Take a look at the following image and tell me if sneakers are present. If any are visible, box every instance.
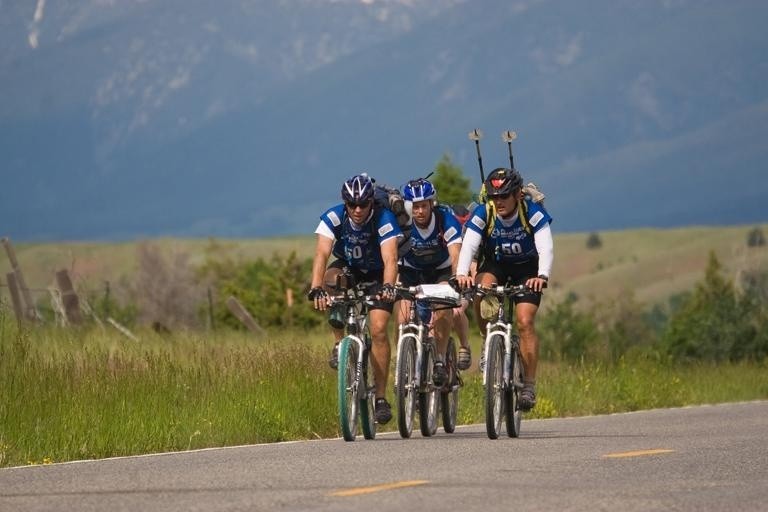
[432,361,446,387]
[521,387,536,404]
[375,398,392,424]
[458,345,472,370]
[330,342,339,368]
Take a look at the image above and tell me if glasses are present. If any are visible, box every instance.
[489,195,509,200]
[348,201,368,209]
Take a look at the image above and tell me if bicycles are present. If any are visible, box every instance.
[382,282,461,439]
[450,275,550,439]
[314,291,393,442]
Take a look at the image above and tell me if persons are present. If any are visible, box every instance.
[390,178,465,395]
[415,267,471,372]
[310,176,403,425]
[454,170,553,407]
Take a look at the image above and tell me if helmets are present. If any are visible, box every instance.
[403,178,436,202]
[342,176,374,205]
[486,168,521,195]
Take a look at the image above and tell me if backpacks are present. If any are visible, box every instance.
[342,185,413,258]
